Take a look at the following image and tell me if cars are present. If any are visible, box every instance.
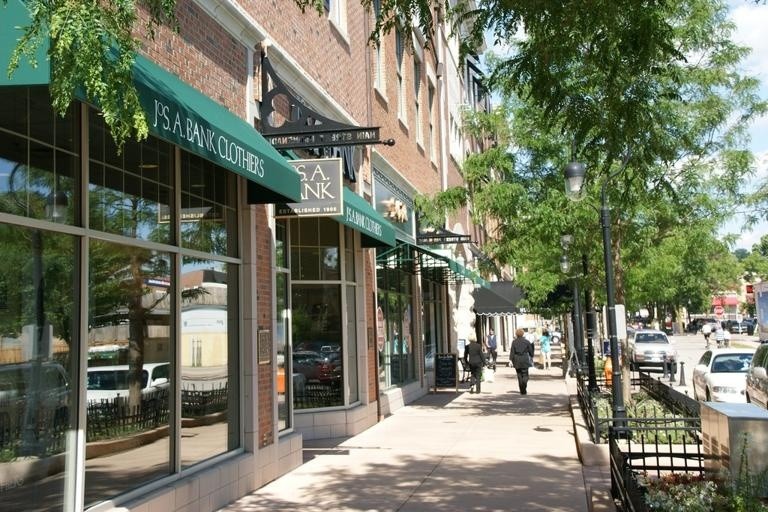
[628,329,677,374]
[692,349,756,404]
[687,319,717,333]
[517,326,562,344]
[293,339,342,387]
[86,358,169,409]
[0,362,70,438]
[746,340,768,411]
[730,323,749,334]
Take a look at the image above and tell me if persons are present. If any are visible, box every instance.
[509,329,533,394]
[464,334,489,393]
[701,322,730,348]
[522,328,534,351]
[539,328,551,369]
[486,328,498,370]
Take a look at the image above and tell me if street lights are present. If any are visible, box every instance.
[562,127,632,439]
[560,229,601,392]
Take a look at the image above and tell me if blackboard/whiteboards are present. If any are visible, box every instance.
[434,353,459,388]
